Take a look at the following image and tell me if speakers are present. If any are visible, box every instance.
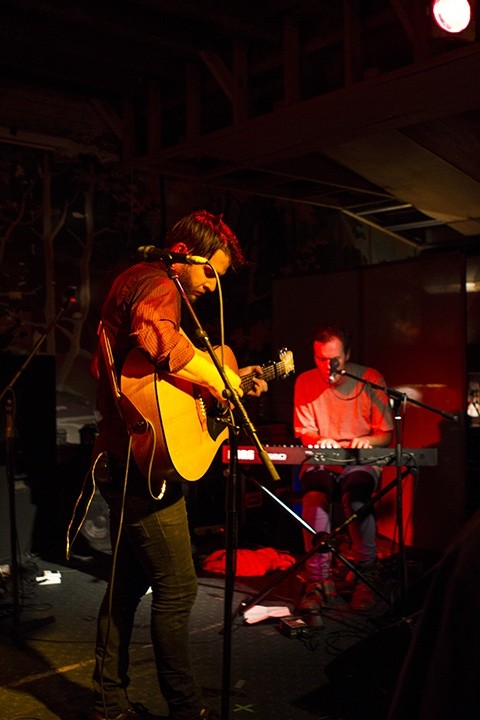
[0,346,114,565]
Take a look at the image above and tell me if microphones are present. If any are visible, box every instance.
[330,358,340,377]
[137,246,208,265]
[63,286,77,303]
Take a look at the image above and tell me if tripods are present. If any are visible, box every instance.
[0,297,70,648]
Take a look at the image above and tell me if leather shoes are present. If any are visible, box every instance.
[300,592,323,610]
[352,572,376,610]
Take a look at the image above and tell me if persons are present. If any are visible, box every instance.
[293,329,394,614]
[91,212,267,720]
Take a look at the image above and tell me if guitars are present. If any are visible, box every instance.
[118,340,297,485]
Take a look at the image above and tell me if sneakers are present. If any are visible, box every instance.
[196,706,224,720]
[94,702,152,720]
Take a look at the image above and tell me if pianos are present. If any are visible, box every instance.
[221,442,433,613]
